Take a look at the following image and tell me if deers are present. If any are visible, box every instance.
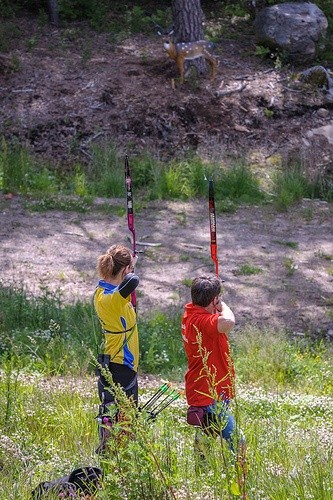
[156,29,217,89]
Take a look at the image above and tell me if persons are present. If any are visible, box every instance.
[180,272,248,478]
[91,242,143,460]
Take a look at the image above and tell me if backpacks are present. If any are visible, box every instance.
[32,462,101,497]
